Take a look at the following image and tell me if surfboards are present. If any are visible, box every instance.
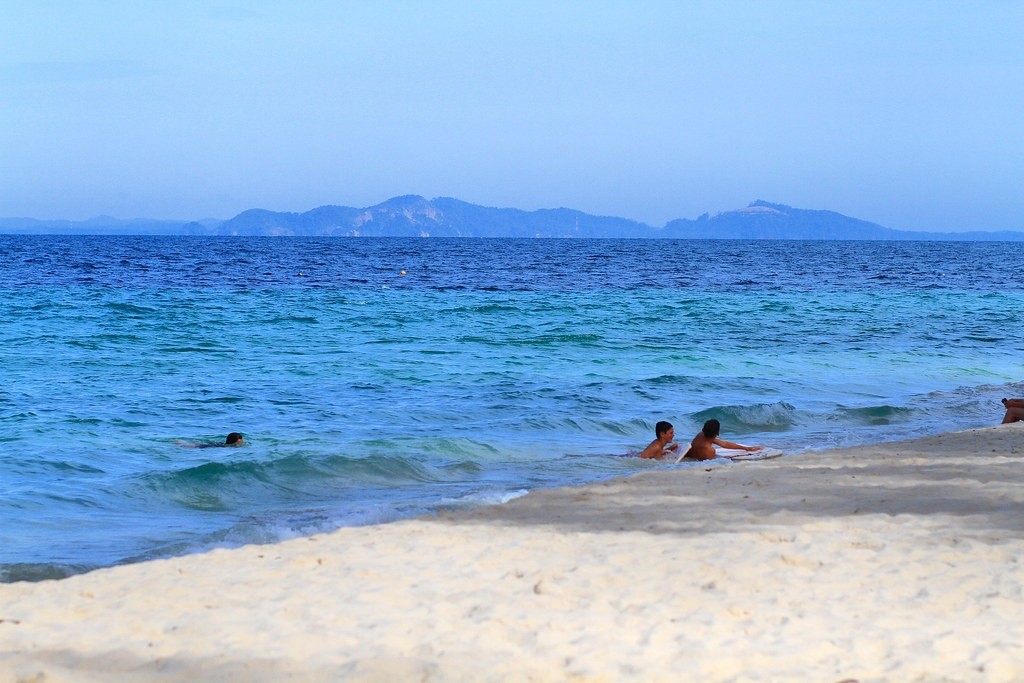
[715,446,783,460]
[666,443,692,466]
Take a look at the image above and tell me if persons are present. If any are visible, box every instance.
[686,419,760,461]
[226,433,242,443]
[641,421,678,459]
[1002,399,1024,423]
